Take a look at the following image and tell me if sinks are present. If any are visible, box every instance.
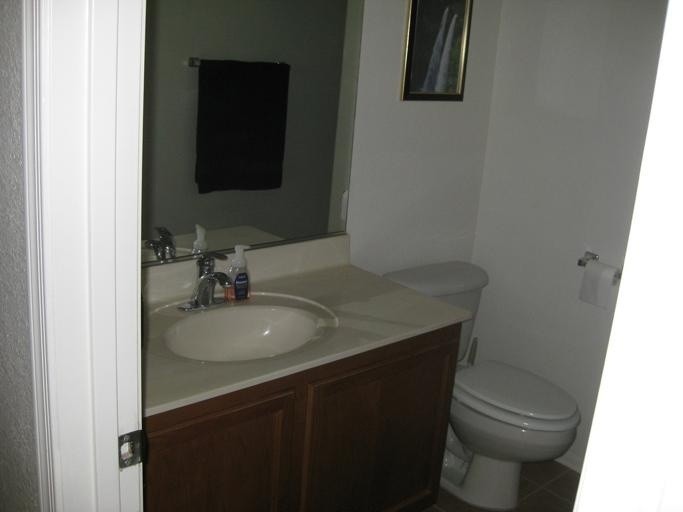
[143,291,340,363]
[140,245,194,265]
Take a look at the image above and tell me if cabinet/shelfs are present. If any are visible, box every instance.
[144,323,460,511]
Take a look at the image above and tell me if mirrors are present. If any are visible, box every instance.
[142,0,366,271]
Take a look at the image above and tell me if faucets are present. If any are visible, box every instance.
[192,250,233,304]
[144,227,177,259]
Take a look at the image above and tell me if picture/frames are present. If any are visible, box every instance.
[400,0,472,101]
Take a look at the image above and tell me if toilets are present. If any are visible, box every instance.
[384,263,581,511]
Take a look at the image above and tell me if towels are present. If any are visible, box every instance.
[196,59,291,195]
